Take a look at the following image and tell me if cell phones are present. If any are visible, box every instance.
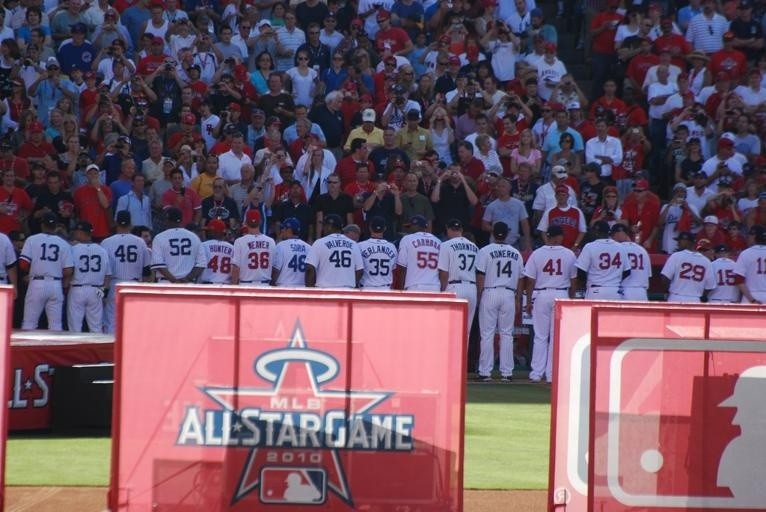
[180,186,185,197]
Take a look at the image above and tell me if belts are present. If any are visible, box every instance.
[447,279,604,294]
[0,274,272,288]
[358,282,394,288]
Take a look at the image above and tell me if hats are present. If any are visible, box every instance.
[0,0,765,252]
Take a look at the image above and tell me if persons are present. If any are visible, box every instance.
[1,1,766,385]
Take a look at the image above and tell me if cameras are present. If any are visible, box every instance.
[114,144,124,148]
[263,178,272,182]
[507,97,513,102]
[386,185,393,189]
[689,109,699,114]
[211,84,220,90]
[396,97,405,106]
[98,88,109,104]
[450,170,460,177]
[23,56,32,66]
[632,128,640,133]
